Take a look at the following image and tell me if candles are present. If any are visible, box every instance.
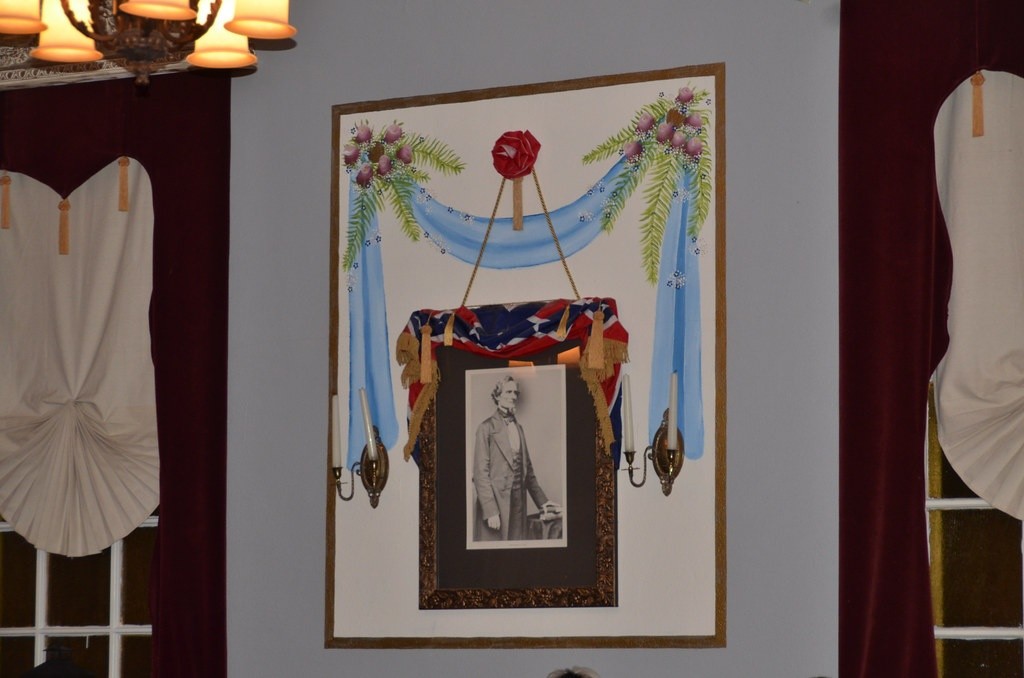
[359,387,377,459]
[667,370,679,449]
[332,394,340,467]
[622,374,635,451]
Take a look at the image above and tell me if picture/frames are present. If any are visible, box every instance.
[400,296,630,609]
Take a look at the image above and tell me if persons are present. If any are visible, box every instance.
[472,376,560,541]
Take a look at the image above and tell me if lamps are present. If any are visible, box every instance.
[0,0,296,85]
